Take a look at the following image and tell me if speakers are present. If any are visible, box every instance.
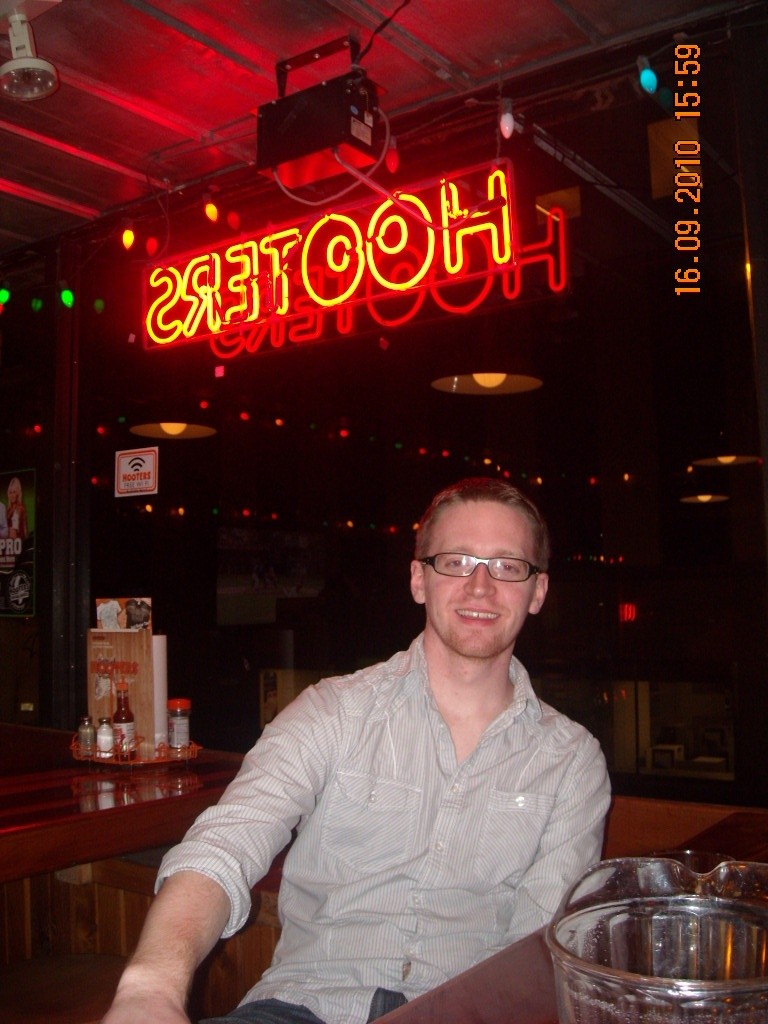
[254,72,378,190]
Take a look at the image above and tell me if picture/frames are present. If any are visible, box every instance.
[0,468,37,619]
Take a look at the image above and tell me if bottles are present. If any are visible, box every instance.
[79,718,95,756]
[113,683,136,760]
[168,699,191,748]
[97,718,114,758]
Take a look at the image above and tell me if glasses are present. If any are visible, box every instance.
[420,551,545,582]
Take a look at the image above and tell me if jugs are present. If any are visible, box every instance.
[544,857,768,1024]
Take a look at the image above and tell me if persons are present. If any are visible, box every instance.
[100,477,612,1024]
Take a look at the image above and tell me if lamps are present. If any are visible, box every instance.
[431,327,543,395]
[674,476,731,505]
[1,8,58,101]
[128,396,218,438]
[687,429,761,468]
